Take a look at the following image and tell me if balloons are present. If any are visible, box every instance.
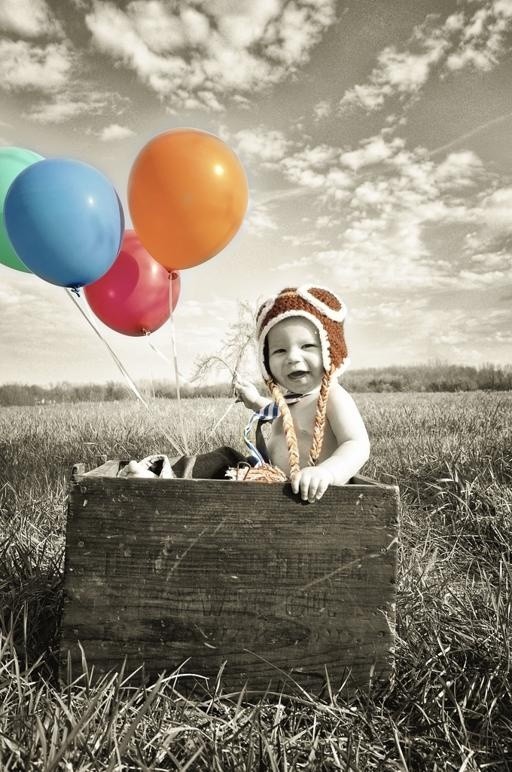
[4,157,125,286]
[84,230,181,336]
[128,130,246,272]
[0,145,46,272]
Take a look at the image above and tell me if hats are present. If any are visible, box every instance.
[256,288,348,383]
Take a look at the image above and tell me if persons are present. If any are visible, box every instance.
[232,285,370,505]
